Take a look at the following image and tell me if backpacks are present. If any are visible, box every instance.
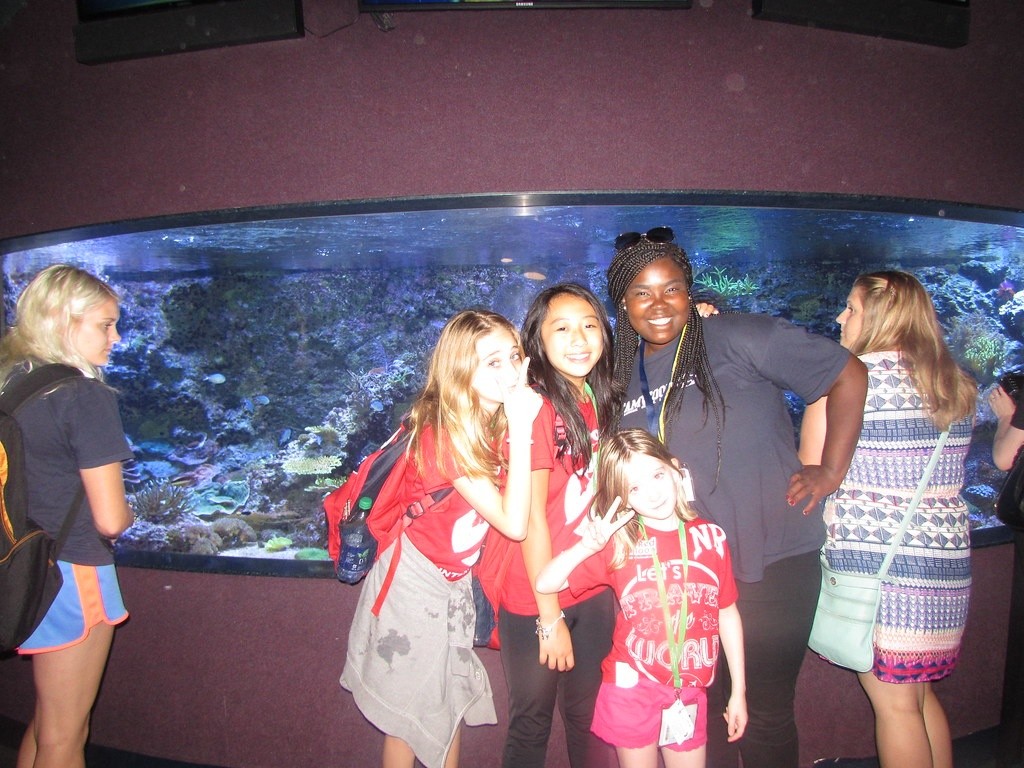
[471,541,502,652]
[323,417,453,584]
[1,363,86,652]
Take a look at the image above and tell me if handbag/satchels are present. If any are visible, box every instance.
[807,555,883,672]
[994,445,1024,534]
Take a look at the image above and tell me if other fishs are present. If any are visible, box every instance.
[279,428,292,445]
[253,395,269,405]
[202,373,226,385]
[370,401,384,412]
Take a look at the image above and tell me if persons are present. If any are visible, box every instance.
[497,283,721,767]
[985,372,1024,767]
[799,270,980,768]
[534,427,749,768]
[339,308,545,767]
[0,261,135,768]
[600,233,870,768]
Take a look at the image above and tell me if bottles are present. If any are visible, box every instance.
[335,496,374,585]
[470,575,491,646]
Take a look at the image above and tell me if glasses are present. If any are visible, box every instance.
[614,226,674,250]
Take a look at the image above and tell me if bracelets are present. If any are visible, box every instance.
[534,608,565,640]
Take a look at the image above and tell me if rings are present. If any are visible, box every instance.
[989,396,995,403]
[994,393,1000,399]
